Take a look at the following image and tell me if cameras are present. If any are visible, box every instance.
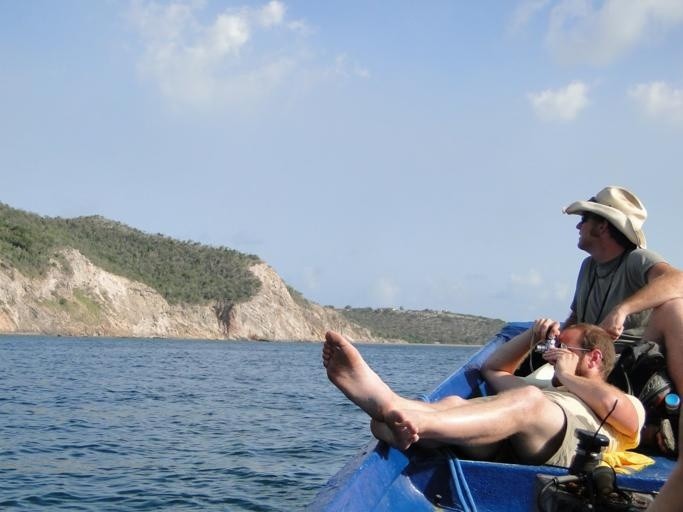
[534,335,557,354]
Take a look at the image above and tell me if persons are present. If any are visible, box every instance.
[563,187,683,453]
[322,318,647,469]
[643,405,683,512]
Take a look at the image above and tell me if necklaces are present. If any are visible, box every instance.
[594,263,617,279]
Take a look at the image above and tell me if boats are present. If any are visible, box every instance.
[298,321,678,512]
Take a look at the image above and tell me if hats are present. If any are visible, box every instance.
[563,186,648,249]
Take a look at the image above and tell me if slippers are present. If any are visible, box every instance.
[646,418,675,457]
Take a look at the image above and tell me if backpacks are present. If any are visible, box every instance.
[607,340,674,417]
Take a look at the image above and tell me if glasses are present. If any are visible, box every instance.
[560,343,592,353]
